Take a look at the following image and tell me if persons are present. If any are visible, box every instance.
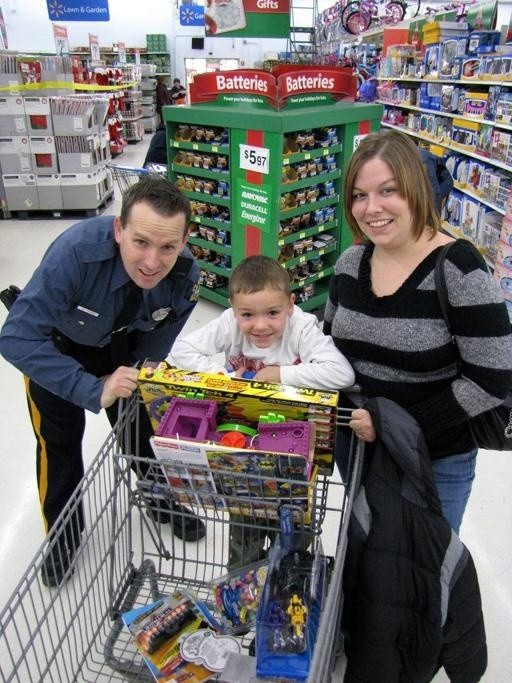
[142,123,167,169]
[0,176,207,588]
[170,78,188,105]
[155,75,172,123]
[169,254,357,658]
[323,127,512,539]
[408,134,454,224]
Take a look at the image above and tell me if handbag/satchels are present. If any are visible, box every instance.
[470,405,512,452]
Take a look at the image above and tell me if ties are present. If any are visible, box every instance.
[111,280,142,342]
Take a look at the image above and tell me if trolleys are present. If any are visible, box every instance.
[2,358,368,681]
[106,160,167,209]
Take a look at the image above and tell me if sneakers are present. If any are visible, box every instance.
[42,534,82,587]
[146,505,206,542]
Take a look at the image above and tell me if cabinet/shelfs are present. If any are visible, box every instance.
[164,76,510,318]
